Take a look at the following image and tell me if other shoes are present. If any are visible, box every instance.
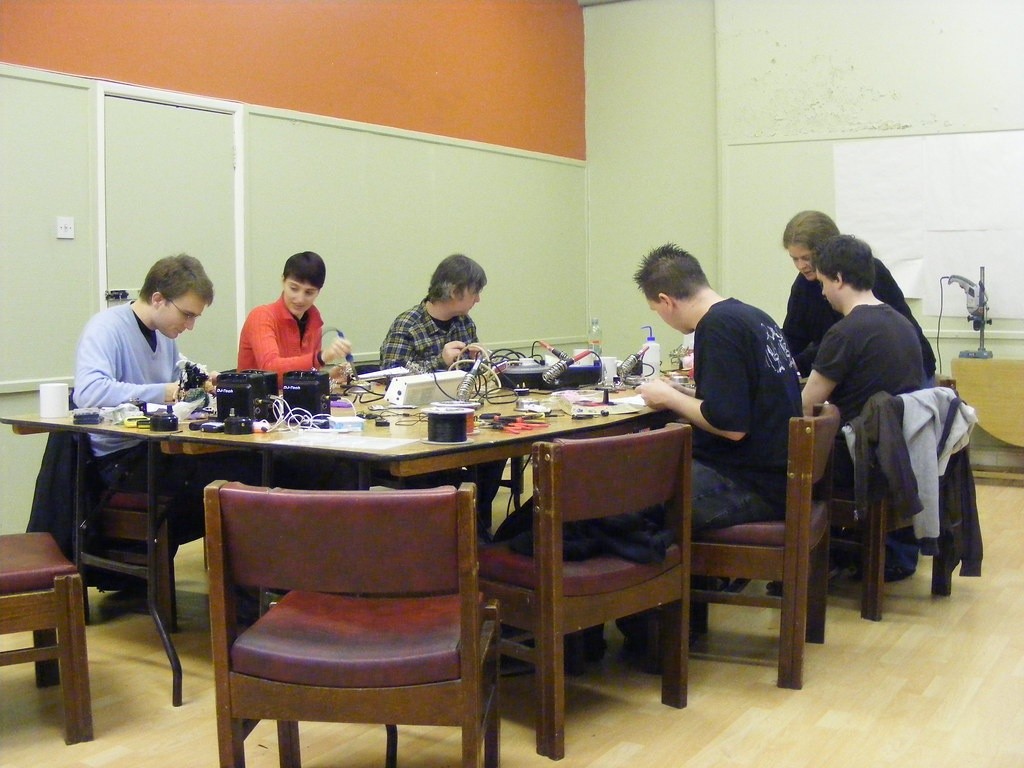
[564,627,609,664]
[618,635,665,674]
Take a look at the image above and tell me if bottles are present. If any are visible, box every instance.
[642,336,661,379]
[587,318,603,356]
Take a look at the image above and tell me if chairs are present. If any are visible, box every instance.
[647,403,841,690]
[23,386,208,632]
[477,422,693,761]
[0,532,94,745]
[204,480,503,768]
[832,376,982,622]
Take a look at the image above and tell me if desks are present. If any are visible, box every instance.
[0,359,812,768]
[950,357,1024,480]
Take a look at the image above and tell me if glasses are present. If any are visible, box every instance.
[169,300,201,320]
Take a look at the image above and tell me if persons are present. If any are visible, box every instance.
[766,234,924,593]
[379,252,508,548]
[73,251,258,624]
[782,211,937,581]
[564,243,802,668]
[236,251,351,389]
